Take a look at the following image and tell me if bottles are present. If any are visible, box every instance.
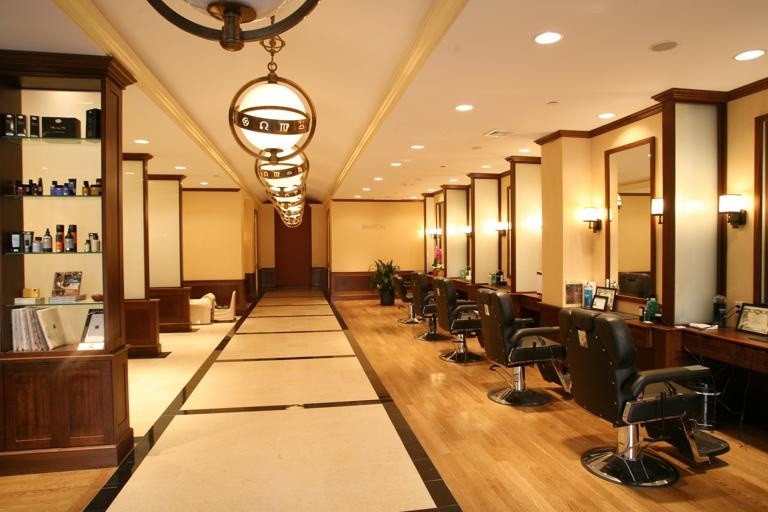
[29,178,101,196]
[25,222,78,253]
[720,307,728,328]
[10,231,21,253]
[654,313,663,322]
[89,232,98,241]
[639,307,644,321]
[84,240,90,252]
[584,282,593,310]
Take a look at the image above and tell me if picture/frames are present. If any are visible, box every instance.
[591,295,609,312]
[596,286,617,312]
[563,280,584,308]
[736,303,768,337]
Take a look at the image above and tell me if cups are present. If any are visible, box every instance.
[91,240,100,253]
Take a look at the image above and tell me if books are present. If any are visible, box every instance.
[11,307,67,351]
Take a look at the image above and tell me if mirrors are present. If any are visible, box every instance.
[435,201,445,272]
[506,185,512,280]
[602,136,657,309]
[751,113,768,309]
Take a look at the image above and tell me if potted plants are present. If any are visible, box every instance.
[367,257,404,307]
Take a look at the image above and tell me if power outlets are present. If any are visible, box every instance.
[733,301,743,312]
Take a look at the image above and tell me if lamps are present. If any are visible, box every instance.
[717,193,748,229]
[228,11,317,166]
[581,207,603,234]
[265,180,306,228]
[255,144,310,197]
[649,197,664,226]
[145,0,321,52]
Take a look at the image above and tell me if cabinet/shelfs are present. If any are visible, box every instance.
[0,48,135,477]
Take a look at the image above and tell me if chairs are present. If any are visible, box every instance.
[556,305,734,491]
[431,275,491,365]
[472,286,577,407]
[408,271,450,343]
[391,274,427,325]
